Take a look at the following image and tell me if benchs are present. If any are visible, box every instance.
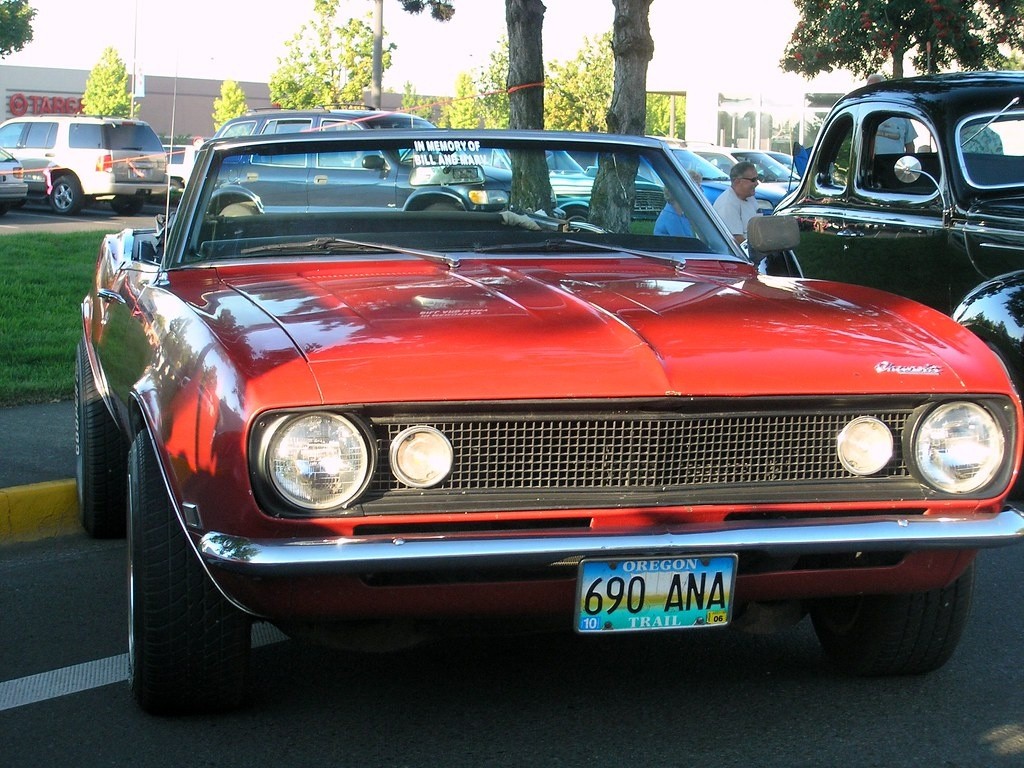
[874,152,1024,190]
[186,205,540,254]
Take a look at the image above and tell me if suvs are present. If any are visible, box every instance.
[0,114,168,215]
[205,104,513,218]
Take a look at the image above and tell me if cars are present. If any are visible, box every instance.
[771,70,1024,407]
[74,128,1024,719]
[0,146,29,215]
[162,145,200,192]
[471,136,801,223]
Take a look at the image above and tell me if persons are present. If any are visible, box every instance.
[961,123,1004,155]
[713,163,791,278]
[653,169,704,239]
[873,117,918,154]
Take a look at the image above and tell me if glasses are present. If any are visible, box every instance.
[740,177,757,182]
